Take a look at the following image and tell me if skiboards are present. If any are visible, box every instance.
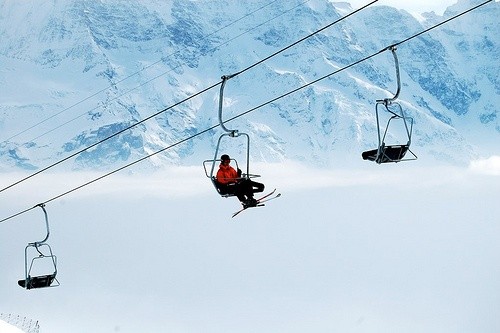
[231,188,282,219]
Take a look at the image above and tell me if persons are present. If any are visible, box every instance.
[215,155,258,208]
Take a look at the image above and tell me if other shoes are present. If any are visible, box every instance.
[242,198,257,209]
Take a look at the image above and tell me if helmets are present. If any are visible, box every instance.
[221,154,230,164]
